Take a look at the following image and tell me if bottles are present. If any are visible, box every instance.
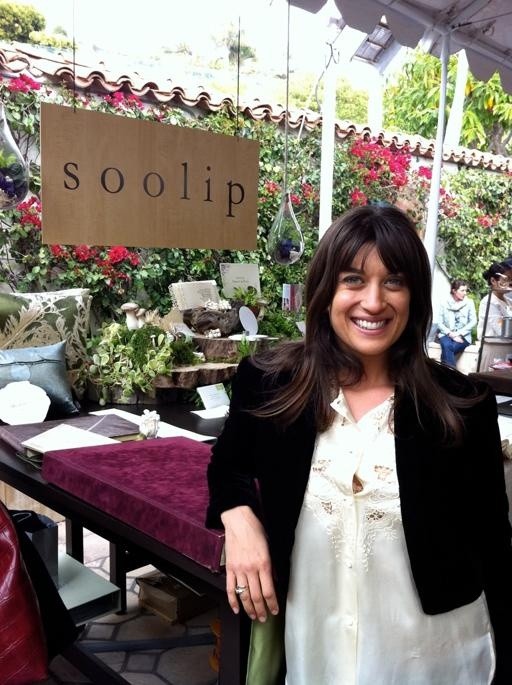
[501,315,512,339]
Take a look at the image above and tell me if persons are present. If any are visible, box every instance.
[201,193,512,684]
[479,262,512,348]
[437,277,477,369]
[500,257,512,303]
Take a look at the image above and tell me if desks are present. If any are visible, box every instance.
[1,399,283,685]
[32,550,129,684]
[470,365,512,425]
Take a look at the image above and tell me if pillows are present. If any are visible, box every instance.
[0,289,95,413]
[0,338,76,419]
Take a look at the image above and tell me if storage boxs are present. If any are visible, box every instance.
[132,569,214,624]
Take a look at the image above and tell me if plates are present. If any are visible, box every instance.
[238,306,259,334]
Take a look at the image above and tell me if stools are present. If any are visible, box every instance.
[428,342,479,373]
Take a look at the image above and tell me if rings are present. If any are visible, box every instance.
[234,585,248,594]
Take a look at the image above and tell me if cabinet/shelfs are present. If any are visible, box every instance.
[474,287,512,374]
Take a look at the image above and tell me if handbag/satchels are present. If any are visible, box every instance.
[9,509,60,591]
